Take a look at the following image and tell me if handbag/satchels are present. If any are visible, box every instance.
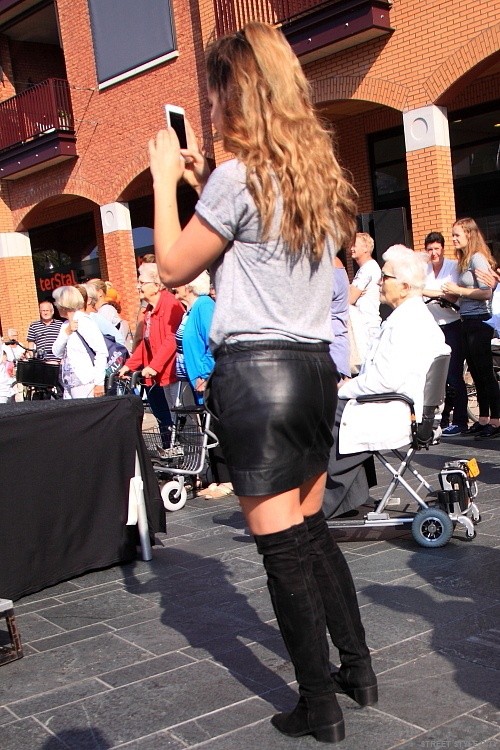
[75,316,130,378]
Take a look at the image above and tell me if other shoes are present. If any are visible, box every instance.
[195,485,216,497]
[204,484,235,500]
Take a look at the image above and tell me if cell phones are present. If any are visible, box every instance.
[165,103,189,153]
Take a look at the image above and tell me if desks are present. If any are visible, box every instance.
[0,395,166,604]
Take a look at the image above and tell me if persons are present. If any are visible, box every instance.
[0,219,500,521]
[148,22,377,743]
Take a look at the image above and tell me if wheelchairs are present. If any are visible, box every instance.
[320,354,481,548]
[103,370,143,396]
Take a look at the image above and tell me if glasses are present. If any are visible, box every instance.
[53,302,59,306]
[136,281,155,285]
[380,272,396,280]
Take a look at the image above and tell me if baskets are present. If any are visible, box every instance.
[140,425,204,471]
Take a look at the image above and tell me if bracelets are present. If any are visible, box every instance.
[460,287,462,297]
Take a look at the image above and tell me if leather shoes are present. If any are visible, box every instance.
[460,421,487,437]
[474,423,500,440]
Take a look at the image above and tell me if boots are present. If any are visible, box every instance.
[252,520,345,743]
[303,507,379,706]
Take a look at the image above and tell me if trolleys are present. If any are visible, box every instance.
[141,380,219,513]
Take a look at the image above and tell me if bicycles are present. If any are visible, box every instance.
[6,339,63,401]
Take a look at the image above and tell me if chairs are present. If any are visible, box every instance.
[36,117,69,134]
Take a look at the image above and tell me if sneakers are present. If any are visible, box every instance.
[441,424,462,436]
